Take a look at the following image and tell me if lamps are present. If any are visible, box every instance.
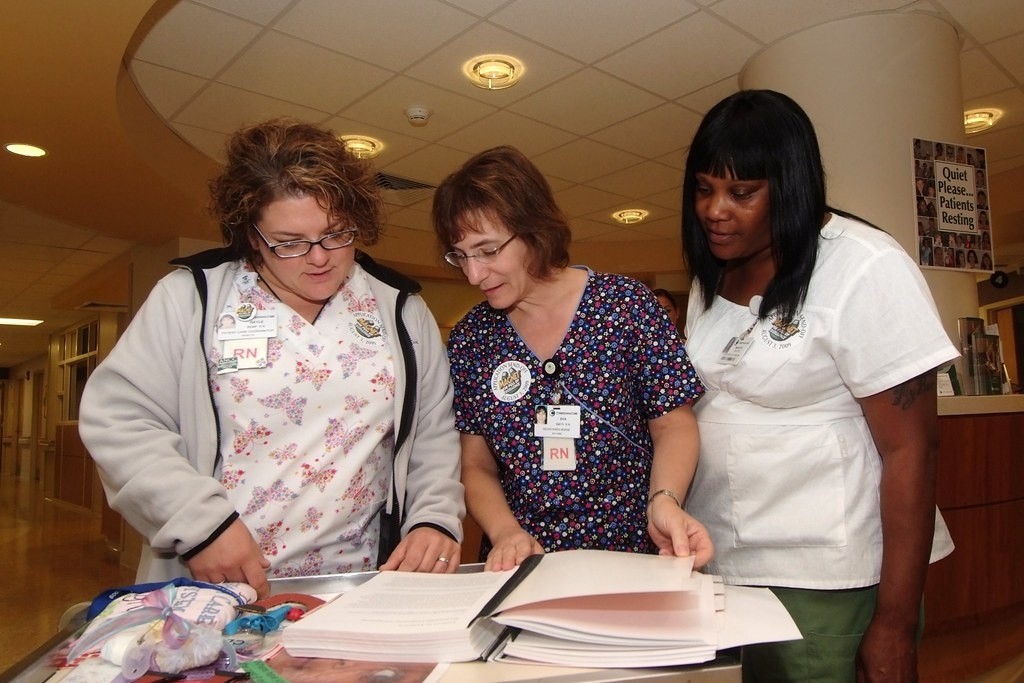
[337,135,385,159]
[964,108,1004,134]
[613,209,648,224]
[462,54,525,90]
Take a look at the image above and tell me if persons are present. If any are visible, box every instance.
[680,89,954,683]
[650,288,680,333]
[912,138,994,271]
[430,146,714,573]
[79,120,467,601]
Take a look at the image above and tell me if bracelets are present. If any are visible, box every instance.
[645,490,681,511]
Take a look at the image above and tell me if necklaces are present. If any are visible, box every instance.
[248,252,341,326]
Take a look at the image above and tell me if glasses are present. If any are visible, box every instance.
[443,233,517,267]
[251,222,359,259]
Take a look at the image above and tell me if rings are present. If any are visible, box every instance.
[437,557,449,566]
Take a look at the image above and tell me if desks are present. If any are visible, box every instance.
[0,563,743,683]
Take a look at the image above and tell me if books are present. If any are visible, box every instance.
[278,551,804,683]
